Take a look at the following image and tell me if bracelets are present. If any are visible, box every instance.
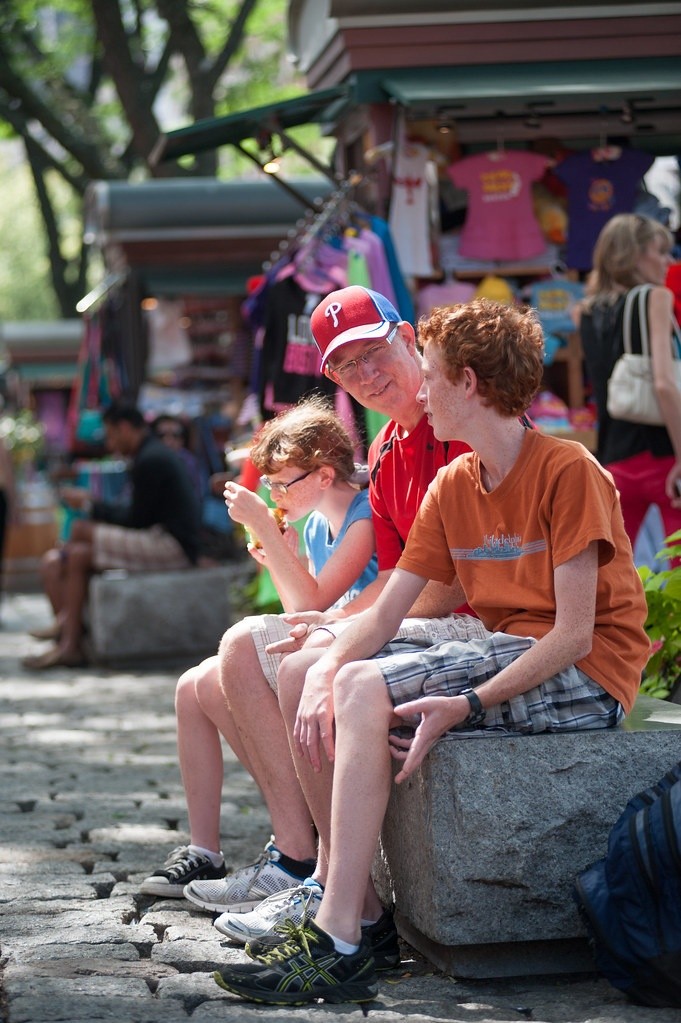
[312,627,336,638]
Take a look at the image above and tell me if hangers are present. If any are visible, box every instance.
[363,135,447,165]
[592,120,621,163]
[489,121,558,167]
[522,258,588,298]
[291,182,369,282]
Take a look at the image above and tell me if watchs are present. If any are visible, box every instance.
[460,688,486,726]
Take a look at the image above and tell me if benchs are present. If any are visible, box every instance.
[366,688,681,978]
[77,568,231,677]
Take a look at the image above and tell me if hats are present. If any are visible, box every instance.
[309,285,402,373]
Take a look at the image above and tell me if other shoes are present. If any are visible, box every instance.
[21,646,82,668]
[28,620,63,645]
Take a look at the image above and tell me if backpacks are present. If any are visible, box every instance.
[570,760,680,1007]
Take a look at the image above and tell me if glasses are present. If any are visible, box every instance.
[157,429,183,438]
[328,325,399,380]
[260,469,315,493]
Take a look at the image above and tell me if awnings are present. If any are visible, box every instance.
[378,57,681,108]
[149,87,349,216]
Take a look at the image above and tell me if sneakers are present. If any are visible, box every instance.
[214,917,378,1005]
[139,846,226,898]
[182,853,306,912]
[214,875,325,942]
[246,904,400,971]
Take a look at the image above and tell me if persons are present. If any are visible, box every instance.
[573,212,681,570]
[22,404,204,671]
[43,415,205,617]
[183,285,535,944]
[139,404,379,897]
[214,298,652,1006]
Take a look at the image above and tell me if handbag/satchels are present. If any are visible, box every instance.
[606,283,681,424]
